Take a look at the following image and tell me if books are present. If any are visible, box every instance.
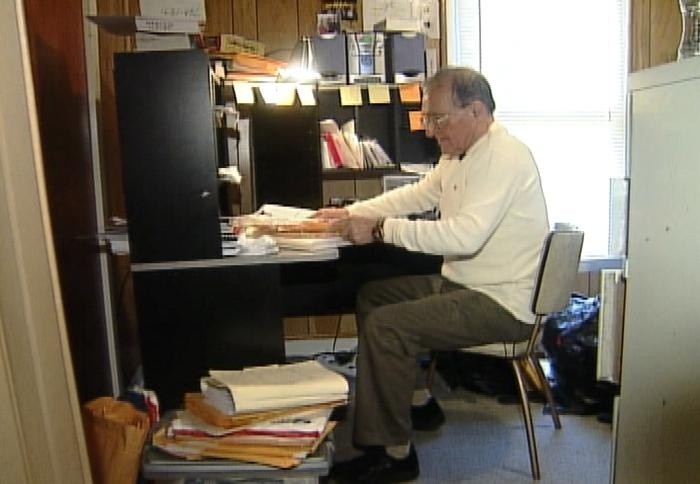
[207,51,280,82]
[95,217,127,241]
[218,203,353,257]
[320,118,392,170]
[151,359,351,471]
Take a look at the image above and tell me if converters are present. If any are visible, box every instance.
[336,352,354,365]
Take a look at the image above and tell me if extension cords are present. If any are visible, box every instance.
[318,354,356,378]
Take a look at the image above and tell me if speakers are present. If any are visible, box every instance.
[308,30,347,83]
[390,30,426,86]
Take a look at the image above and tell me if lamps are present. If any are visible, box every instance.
[263,37,323,87]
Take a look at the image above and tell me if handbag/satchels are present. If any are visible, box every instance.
[81,396,151,484]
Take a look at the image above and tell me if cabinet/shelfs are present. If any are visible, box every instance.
[110,35,451,269]
[589,54,698,481]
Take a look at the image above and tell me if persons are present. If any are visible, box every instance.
[307,67,550,483]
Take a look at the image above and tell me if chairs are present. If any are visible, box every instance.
[454,229,588,480]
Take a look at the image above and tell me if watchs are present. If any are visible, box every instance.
[372,216,384,244]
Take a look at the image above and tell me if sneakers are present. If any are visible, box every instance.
[410,396,445,432]
[319,441,420,484]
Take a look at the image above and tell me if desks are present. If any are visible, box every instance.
[132,218,447,414]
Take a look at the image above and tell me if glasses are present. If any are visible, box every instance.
[419,114,450,129]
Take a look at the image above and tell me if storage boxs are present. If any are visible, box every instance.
[142,442,333,483]
